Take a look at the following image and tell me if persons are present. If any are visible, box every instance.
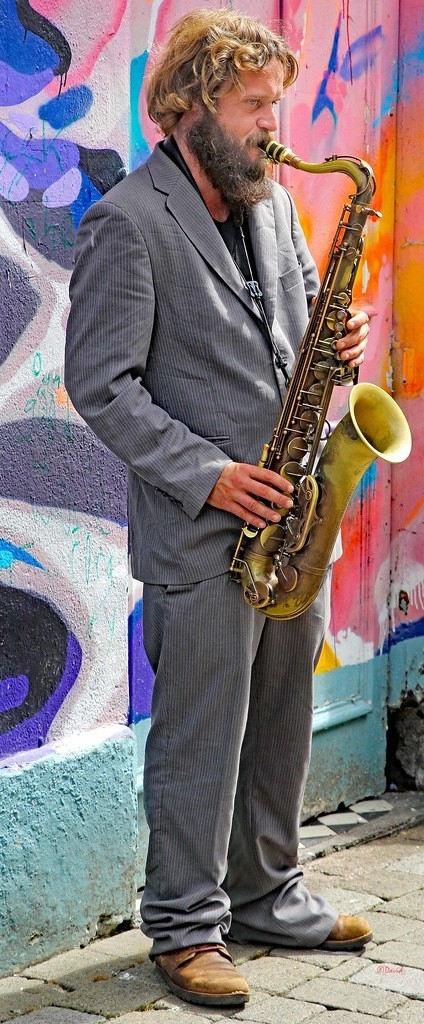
[64,9,374,1005]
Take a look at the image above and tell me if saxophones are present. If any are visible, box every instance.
[222,138,413,619]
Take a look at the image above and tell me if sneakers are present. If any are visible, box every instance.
[152,943,251,1005]
[316,912,374,948]
